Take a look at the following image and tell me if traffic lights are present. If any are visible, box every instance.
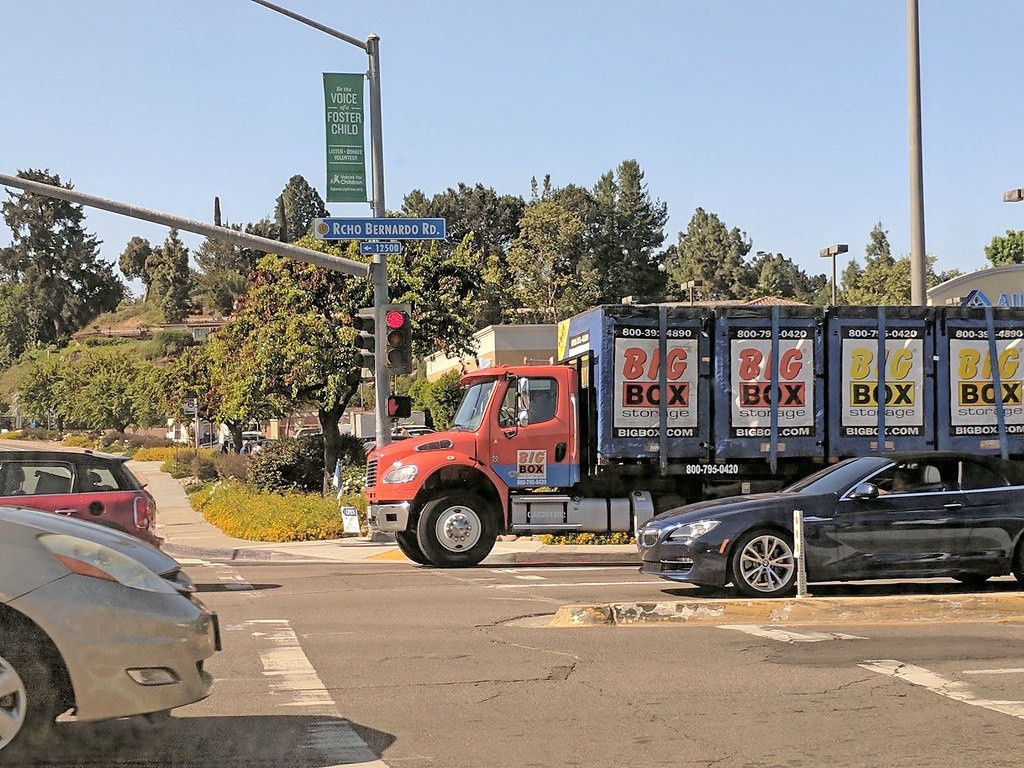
[379,303,412,374]
[352,307,376,379]
[388,395,413,419]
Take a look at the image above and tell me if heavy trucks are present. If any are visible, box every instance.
[365,292,1024,569]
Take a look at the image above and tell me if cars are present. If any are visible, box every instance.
[391,423,436,440]
[240,426,322,457]
[0,442,164,552]
[0,504,223,768]
[634,450,1024,599]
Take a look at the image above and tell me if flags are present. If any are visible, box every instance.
[332,464,345,500]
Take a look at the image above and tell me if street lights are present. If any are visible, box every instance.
[681,280,703,306]
[820,244,849,306]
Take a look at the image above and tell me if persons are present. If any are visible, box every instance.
[874,464,922,495]
[239,440,262,455]
